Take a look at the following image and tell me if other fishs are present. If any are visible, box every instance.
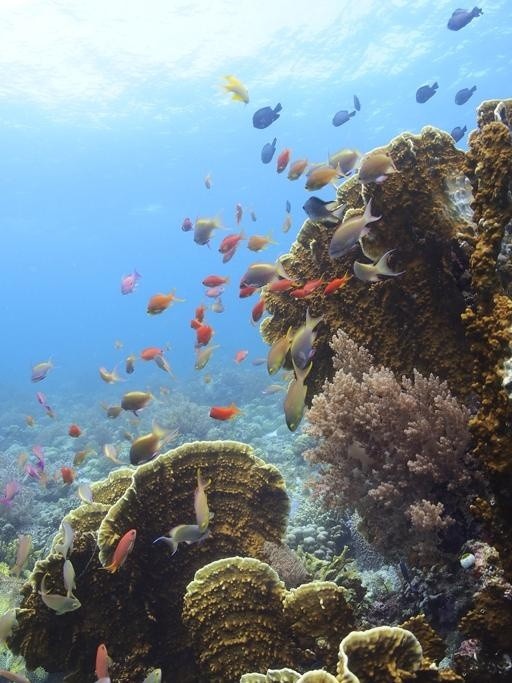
[178,73,406,432]
[0,340,216,639]
